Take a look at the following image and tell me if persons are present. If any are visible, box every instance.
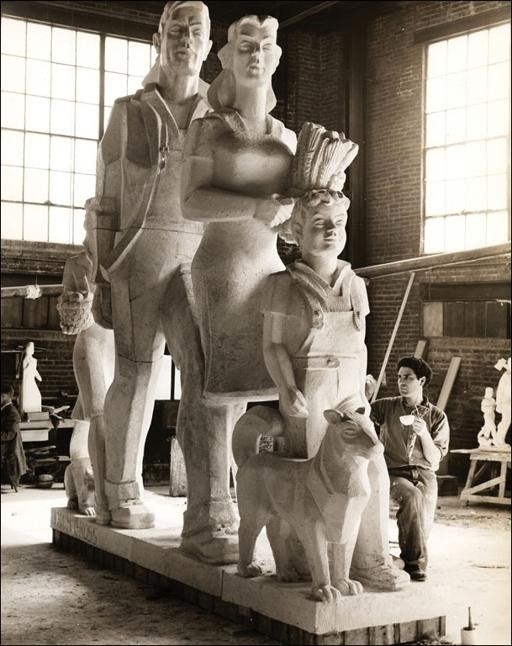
[1,384,30,486]
[366,353,453,582]
[494,357,511,450]
[22,340,44,416]
[479,387,499,439]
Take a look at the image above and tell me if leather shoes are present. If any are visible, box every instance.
[403,565,426,581]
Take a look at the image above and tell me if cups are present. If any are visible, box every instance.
[399,415,415,426]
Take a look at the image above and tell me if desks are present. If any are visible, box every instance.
[461,452,512,508]
